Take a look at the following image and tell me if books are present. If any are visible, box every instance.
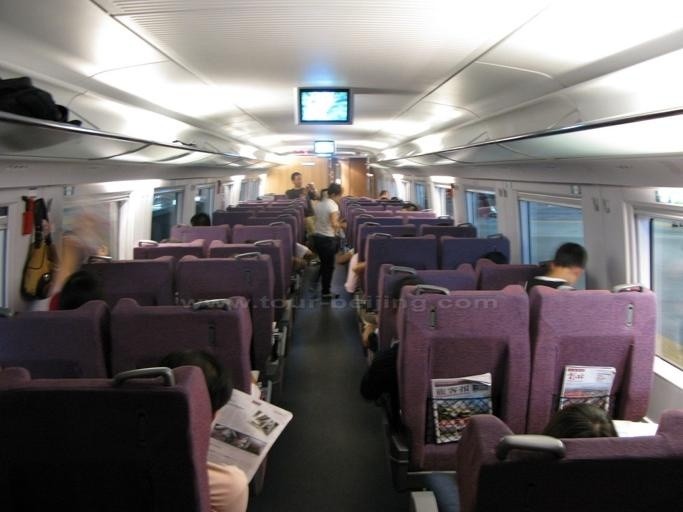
[429,371,494,444]
[560,364,617,414]
[207,387,294,486]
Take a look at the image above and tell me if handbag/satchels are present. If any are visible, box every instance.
[22,200,56,299]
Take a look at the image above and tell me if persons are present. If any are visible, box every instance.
[379,189,389,198]
[403,204,415,211]
[283,172,366,300]
[195,304,262,400]
[422,403,617,511]
[60,271,100,309]
[191,214,212,227]
[170,352,248,512]
[359,344,398,415]
[362,324,377,346]
[525,242,588,290]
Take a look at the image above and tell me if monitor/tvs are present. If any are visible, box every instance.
[314,141,335,154]
[317,154,332,158]
[297,86,353,125]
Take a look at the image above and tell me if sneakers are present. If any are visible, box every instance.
[321,292,340,300]
[309,287,318,299]
